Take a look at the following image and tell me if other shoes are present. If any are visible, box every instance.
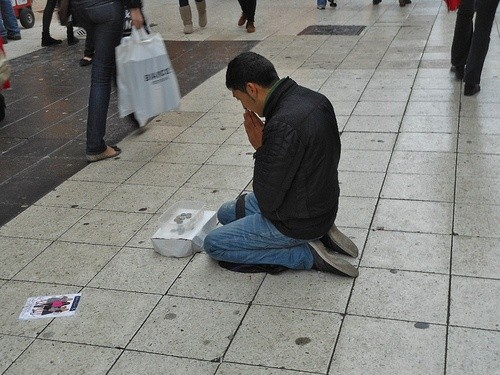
[86,145,122,162]
[317,6,325,10]
[372,0,381,4]
[463,83,480,96]
[307,240,359,278]
[3,33,21,44]
[67,26,79,46]
[79,56,94,67]
[246,21,255,32]
[450,65,464,79]
[320,222,358,258]
[399,0,411,8]
[238,15,246,26]
[42,31,63,46]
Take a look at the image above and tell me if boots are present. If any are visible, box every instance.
[180,5,192,34]
[197,0,207,27]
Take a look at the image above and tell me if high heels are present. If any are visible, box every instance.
[0,93,6,121]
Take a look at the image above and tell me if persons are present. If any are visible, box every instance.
[237,0,258,33]
[203,52,361,279]
[373,0,411,7]
[30,296,74,317]
[450,0,500,96]
[1,0,150,161]
[316,0,337,10]
[178,0,207,34]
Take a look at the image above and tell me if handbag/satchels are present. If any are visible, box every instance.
[115,24,181,128]
[218,193,291,275]
[0,34,12,92]
[445,0,461,12]
